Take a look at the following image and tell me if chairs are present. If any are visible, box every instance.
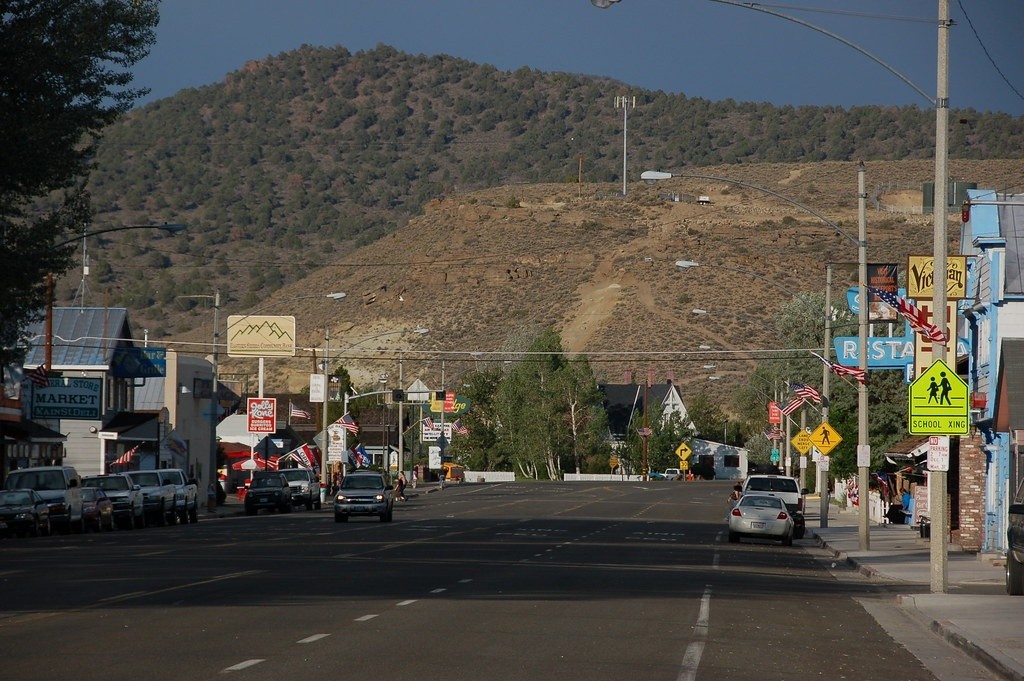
[746,500,754,506]
[771,480,784,490]
[751,479,765,490]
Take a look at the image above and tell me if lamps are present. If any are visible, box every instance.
[178,385,191,395]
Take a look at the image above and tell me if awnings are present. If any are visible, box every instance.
[4,418,67,442]
[98,413,159,440]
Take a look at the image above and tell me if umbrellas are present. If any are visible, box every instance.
[232,458,276,481]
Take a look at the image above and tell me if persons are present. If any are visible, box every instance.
[883,487,911,524]
[412,466,419,488]
[400,471,408,501]
[394,479,405,502]
[438,467,446,489]
[333,471,343,490]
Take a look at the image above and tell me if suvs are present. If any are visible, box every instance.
[333,472,394,523]
[244,470,293,515]
[281,468,322,511]
[741,473,809,539]
[123,470,177,528]
[85,473,146,530]
[157,469,198,524]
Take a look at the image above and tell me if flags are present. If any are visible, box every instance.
[26,366,48,388]
[356,446,371,468]
[866,286,947,342]
[335,413,359,437]
[877,474,889,496]
[790,382,823,404]
[111,445,140,465]
[451,419,468,434]
[348,446,362,469]
[764,426,786,439]
[820,357,865,385]
[290,402,311,420]
[162,431,187,459]
[421,418,433,429]
[290,445,316,476]
[775,398,805,416]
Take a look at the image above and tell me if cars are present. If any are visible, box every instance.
[0,488,51,537]
[728,494,794,546]
[81,486,115,532]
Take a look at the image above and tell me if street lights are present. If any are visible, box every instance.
[640,170,873,550]
[319,328,431,502]
[208,292,347,508]
[675,261,831,527]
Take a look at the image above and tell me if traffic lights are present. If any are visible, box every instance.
[392,388,404,402]
[436,390,446,400]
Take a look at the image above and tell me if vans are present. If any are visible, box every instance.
[7,466,84,534]
[664,468,684,481]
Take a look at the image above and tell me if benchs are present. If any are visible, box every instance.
[900,510,912,516]
